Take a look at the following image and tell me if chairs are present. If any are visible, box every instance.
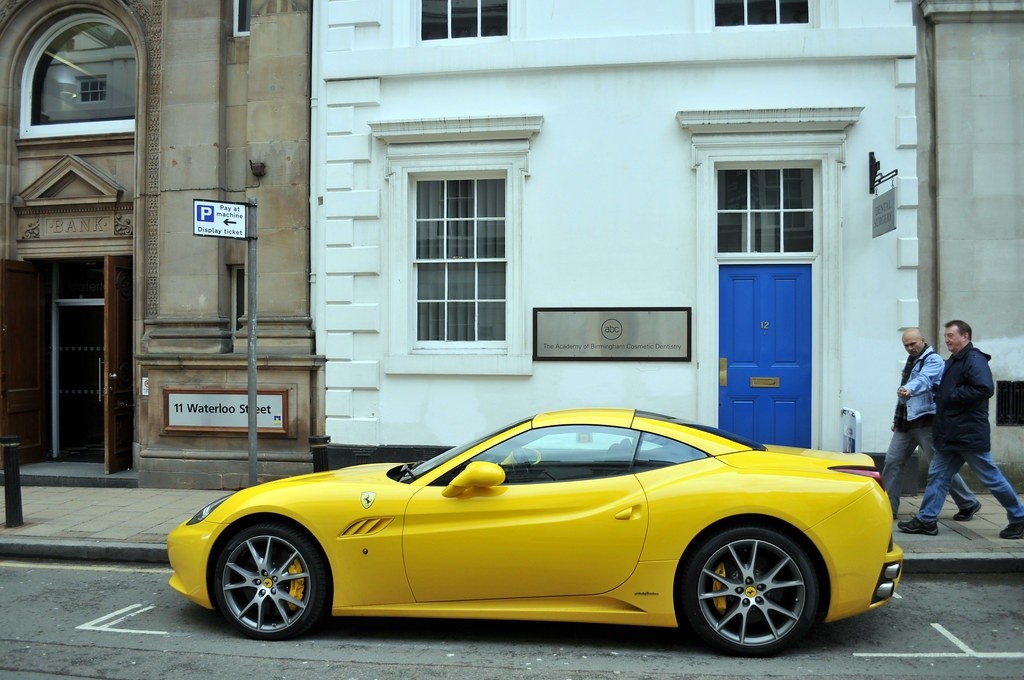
[602,439,634,472]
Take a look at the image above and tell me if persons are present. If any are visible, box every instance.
[881,327,982,521]
[897,320,1024,539]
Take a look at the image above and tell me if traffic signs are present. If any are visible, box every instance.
[192,199,253,243]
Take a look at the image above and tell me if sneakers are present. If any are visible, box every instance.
[953,501,981,521]
[999,520,1024,538]
[898,516,938,535]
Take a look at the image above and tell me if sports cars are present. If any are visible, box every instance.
[166,407,903,661]
